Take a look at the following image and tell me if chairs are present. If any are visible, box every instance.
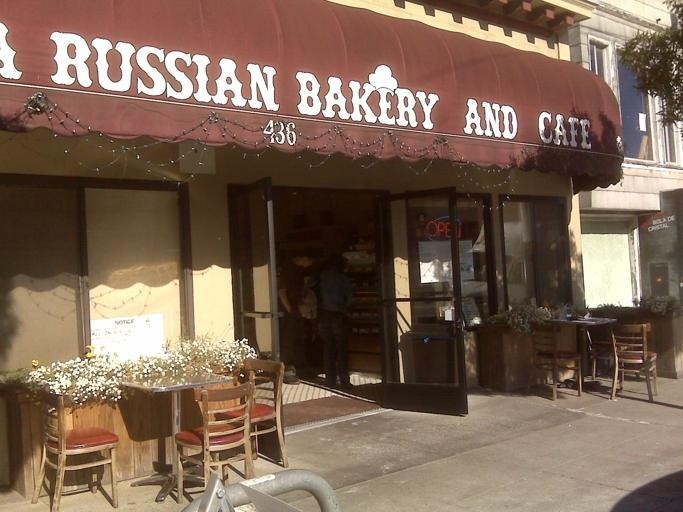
[581,320,630,383]
[215,359,290,473]
[523,323,584,401]
[32,392,118,512]
[608,323,660,403]
[175,381,255,507]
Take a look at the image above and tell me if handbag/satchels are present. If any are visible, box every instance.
[297,288,318,320]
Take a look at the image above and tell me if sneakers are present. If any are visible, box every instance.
[283,367,349,388]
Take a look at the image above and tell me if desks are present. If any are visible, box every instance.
[120,373,238,503]
[560,317,617,386]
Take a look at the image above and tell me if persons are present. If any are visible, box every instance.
[316,252,359,390]
[275,248,316,386]
